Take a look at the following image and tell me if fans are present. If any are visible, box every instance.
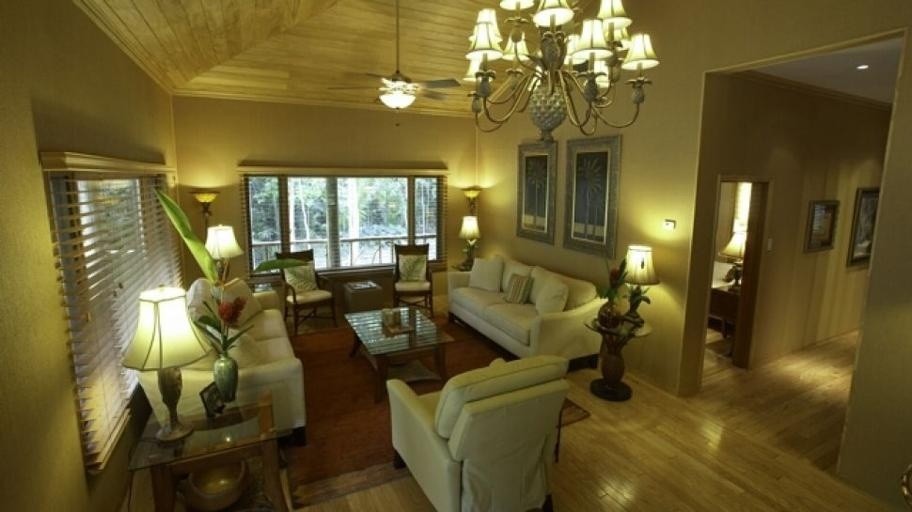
[325,1,460,99]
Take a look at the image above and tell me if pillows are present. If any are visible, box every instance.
[283,260,318,294]
[467,253,503,294]
[503,272,535,305]
[534,276,568,314]
[221,277,263,328]
[204,323,267,367]
[398,254,427,281]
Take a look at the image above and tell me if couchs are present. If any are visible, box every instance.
[139,278,307,448]
[446,253,609,370]
[386,357,566,510]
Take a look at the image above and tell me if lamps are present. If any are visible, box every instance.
[463,0,661,141]
[462,186,482,213]
[375,77,426,129]
[118,289,214,441]
[457,215,480,271]
[722,230,748,290]
[623,247,660,327]
[188,190,221,220]
[205,226,246,282]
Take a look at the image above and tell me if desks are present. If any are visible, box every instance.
[581,323,653,402]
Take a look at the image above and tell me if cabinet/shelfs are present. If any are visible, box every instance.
[342,281,383,311]
[126,390,288,512]
[712,285,742,339]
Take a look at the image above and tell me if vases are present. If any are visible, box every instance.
[598,301,622,328]
[212,350,239,402]
[199,382,225,417]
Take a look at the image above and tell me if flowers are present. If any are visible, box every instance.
[155,186,309,355]
[597,254,629,301]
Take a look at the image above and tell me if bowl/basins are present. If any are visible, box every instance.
[188,453,251,512]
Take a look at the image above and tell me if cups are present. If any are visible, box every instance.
[408,305,417,322]
[382,308,401,326]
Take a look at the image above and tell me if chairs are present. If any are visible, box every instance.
[391,242,434,319]
[274,248,338,338]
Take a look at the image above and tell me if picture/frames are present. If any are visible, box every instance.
[846,185,880,269]
[515,141,557,246]
[562,136,623,262]
[803,199,839,254]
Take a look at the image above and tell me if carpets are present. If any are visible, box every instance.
[283,312,592,510]
